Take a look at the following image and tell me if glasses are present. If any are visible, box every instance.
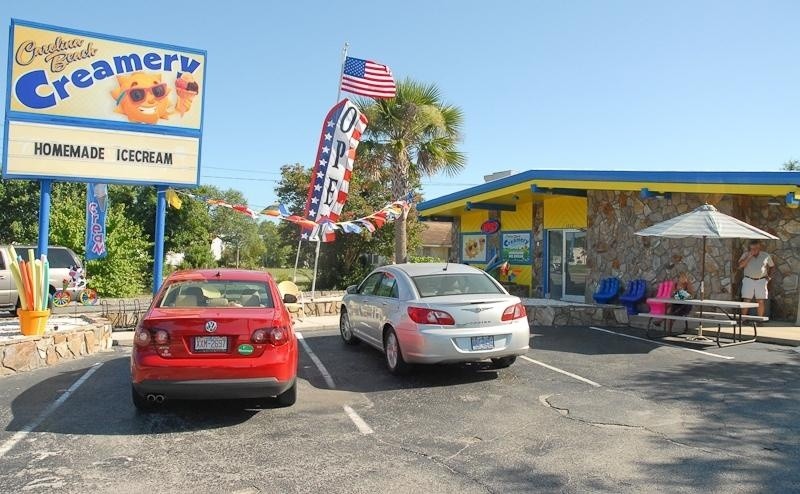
[125,83,167,102]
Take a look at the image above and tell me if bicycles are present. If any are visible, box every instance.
[52,279,97,307]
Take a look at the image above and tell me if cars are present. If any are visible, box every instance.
[131,270,298,409]
[339,262,530,377]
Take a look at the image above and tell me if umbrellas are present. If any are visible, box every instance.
[629,201,780,325]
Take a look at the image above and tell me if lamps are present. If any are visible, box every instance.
[768,198,781,205]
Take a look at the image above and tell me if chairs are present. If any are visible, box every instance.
[279,281,304,322]
[618,279,647,315]
[593,277,619,304]
[646,280,674,313]
[174,291,265,307]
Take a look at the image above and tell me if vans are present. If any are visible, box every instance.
[0,246,86,316]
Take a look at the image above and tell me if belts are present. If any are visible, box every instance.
[745,275,767,280]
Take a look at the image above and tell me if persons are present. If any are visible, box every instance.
[652,270,697,337]
[735,237,776,326]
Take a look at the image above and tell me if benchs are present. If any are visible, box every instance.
[640,313,736,347]
[696,312,769,340]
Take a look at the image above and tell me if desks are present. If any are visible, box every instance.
[651,298,759,343]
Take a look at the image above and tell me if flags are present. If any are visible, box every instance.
[299,98,370,242]
[166,186,414,235]
[340,57,397,100]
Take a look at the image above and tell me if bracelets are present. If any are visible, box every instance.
[768,276,772,280]
